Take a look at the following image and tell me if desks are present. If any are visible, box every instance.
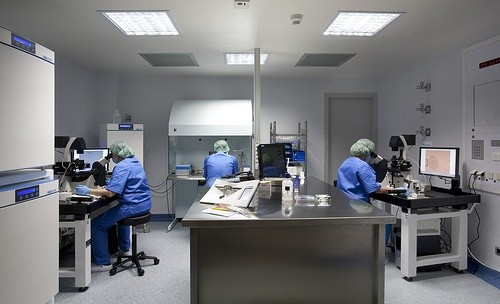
[168,174,246,232]
[59,194,119,292]
[182,176,396,304]
[333,179,480,282]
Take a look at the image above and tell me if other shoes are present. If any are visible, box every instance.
[91,262,113,273]
[117,251,132,257]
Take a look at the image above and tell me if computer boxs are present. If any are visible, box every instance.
[394,234,442,272]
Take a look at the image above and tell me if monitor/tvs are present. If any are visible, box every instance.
[418,147,459,180]
[73,148,110,175]
[257,143,287,179]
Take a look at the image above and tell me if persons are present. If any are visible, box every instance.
[76,139,151,271]
[337,138,392,247]
[203,139,240,182]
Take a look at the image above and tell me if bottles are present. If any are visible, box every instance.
[282,202,292,217]
[113,110,121,123]
[282,173,294,201]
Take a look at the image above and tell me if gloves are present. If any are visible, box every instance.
[76,185,90,196]
[104,178,112,187]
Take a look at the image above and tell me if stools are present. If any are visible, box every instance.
[108,213,159,276]
